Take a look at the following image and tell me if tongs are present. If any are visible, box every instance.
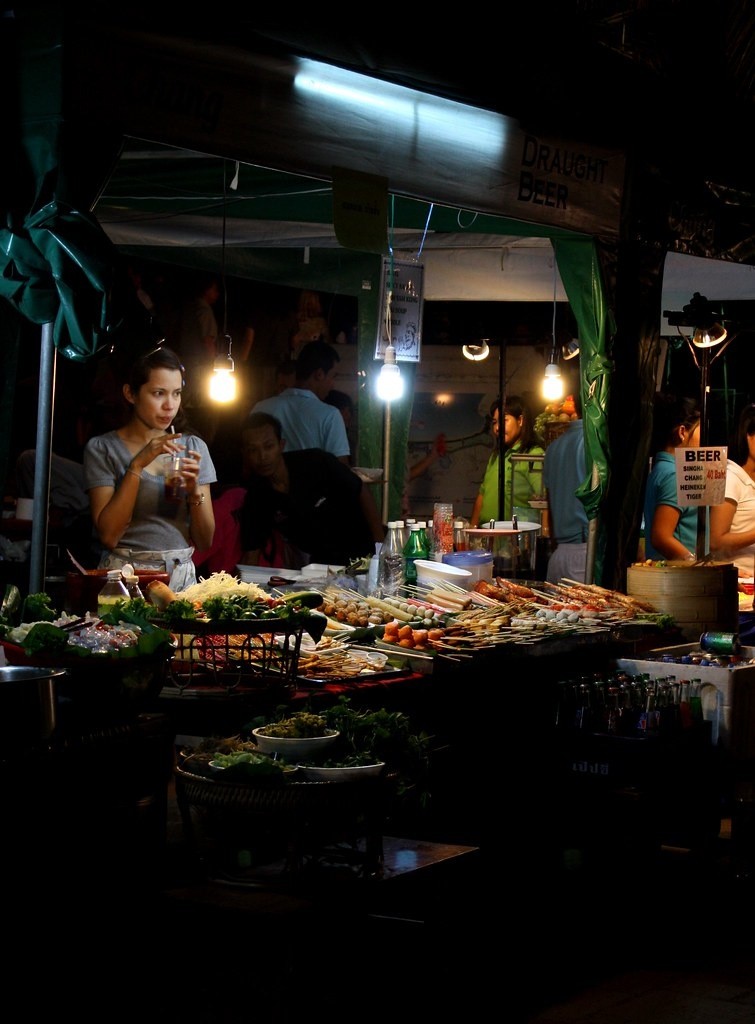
[345,561,369,575]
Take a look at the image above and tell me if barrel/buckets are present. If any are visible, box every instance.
[416,578,468,600]
[465,521,542,579]
[443,552,494,592]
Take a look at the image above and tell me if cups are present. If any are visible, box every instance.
[162,455,190,504]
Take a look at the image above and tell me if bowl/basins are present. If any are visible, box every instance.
[413,559,473,580]
[352,467,383,483]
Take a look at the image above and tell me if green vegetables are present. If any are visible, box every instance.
[211,696,440,813]
[0,581,310,661]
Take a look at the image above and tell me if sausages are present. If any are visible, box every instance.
[427,589,475,610]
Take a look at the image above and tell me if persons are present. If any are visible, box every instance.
[84,347,217,592]
[180,276,219,358]
[191,422,289,573]
[402,445,440,520]
[240,412,382,568]
[544,382,589,582]
[471,395,550,581]
[644,395,710,560]
[291,290,331,343]
[709,403,755,647]
[251,340,353,465]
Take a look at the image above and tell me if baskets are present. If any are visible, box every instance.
[139,610,309,698]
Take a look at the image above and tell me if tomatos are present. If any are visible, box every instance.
[544,395,580,423]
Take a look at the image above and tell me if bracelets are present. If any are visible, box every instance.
[191,493,205,506]
[127,469,142,479]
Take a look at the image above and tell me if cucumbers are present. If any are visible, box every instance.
[285,593,324,610]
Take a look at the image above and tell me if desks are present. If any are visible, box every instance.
[146,834,482,917]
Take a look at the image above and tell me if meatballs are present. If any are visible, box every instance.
[315,597,446,651]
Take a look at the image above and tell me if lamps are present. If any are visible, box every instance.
[457,318,491,362]
[557,327,580,361]
[682,292,727,348]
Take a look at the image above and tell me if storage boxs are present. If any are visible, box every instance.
[609,640,755,756]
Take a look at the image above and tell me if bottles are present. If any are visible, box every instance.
[126,576,145,603]
[428,521,434,544]
[405,519,415,542]
[97,570,131,621]
[377,521,405,600]
[416,521,430,548]
[403,524,429,599]
[549,668,704,741]
[453,521,466,552]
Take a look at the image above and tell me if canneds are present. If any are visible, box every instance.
[699,631,741,654]
